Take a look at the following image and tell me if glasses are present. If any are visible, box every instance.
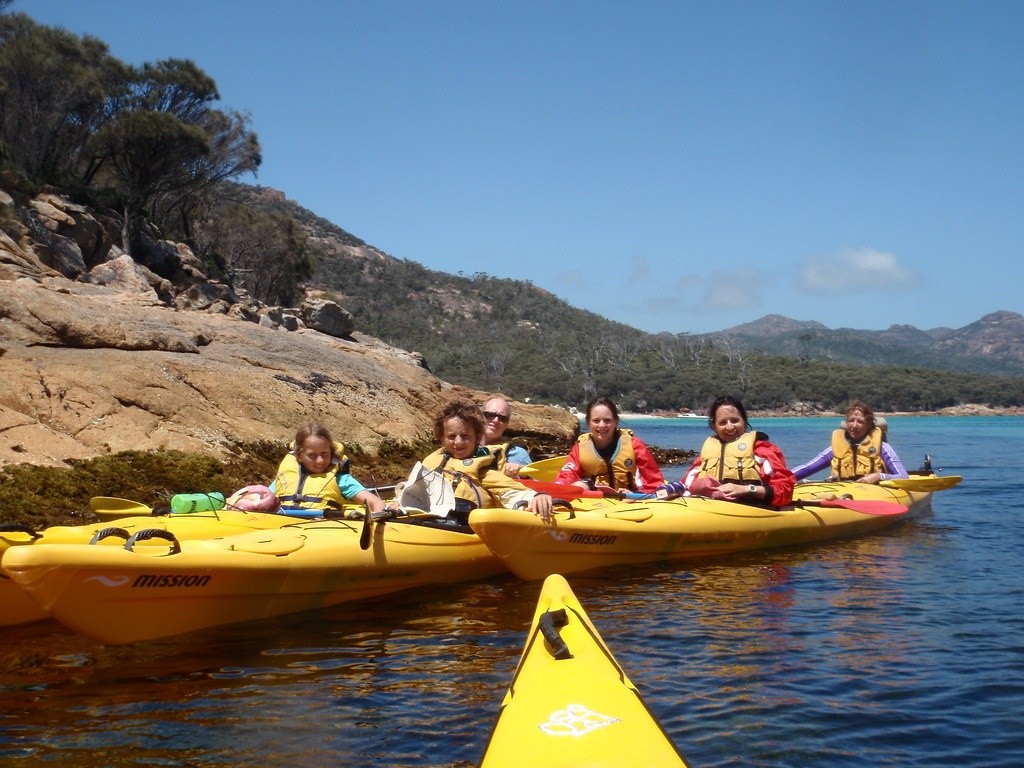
[482,410,510,424]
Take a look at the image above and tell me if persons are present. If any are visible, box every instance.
[552,397,665,493]
[680,395,797,508]
[387,397,554,521]
[268,423,386,514]
[790,399,909,485]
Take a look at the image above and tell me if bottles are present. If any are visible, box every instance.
[655,481,687,501]
[170,490,226,513]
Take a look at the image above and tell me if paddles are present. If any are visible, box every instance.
[784,499,909,515]
[365,455,569,493]
[512,477,656,503]
[90,497,358,523]
[359,499,424,551]
[877,476,961,492]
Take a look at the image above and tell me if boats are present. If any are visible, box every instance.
[482,572,689,768]
[466,456,936,580]
[0,508,515,648]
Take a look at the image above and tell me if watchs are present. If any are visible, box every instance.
[748,484,756,497]
